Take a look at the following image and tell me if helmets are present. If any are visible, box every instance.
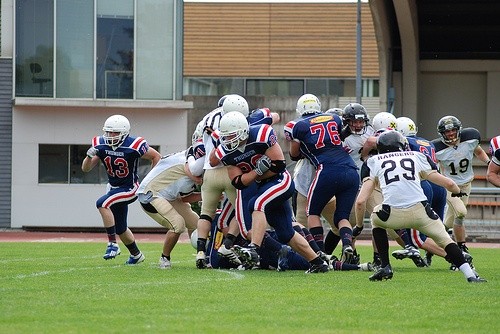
[296,93,321,117]
[102,114,130,142]
[222,94,249,117]
[342,103,370,126]
[191,228,210,250]
[375,129,411,155]
[372,111,397,132]
[218,111,249,140]
[327,107,343,116]
[436,115,463,143]
[397,117,417,137]
[218,94,228,107]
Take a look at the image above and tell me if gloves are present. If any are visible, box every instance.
[451,190,468,198]
[352,225,363,240]
[256,155,271,176]
[86,147,99,159]
[342,145,354,154]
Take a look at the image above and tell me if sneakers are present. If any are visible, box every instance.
[215,245,487,282]
[103,242,121,260]
[124,251,145,265]
[159,256,171,268]
[195,251,206,269]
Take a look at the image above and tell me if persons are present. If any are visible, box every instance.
[135,96,500,284]
[82,114,163,264]
